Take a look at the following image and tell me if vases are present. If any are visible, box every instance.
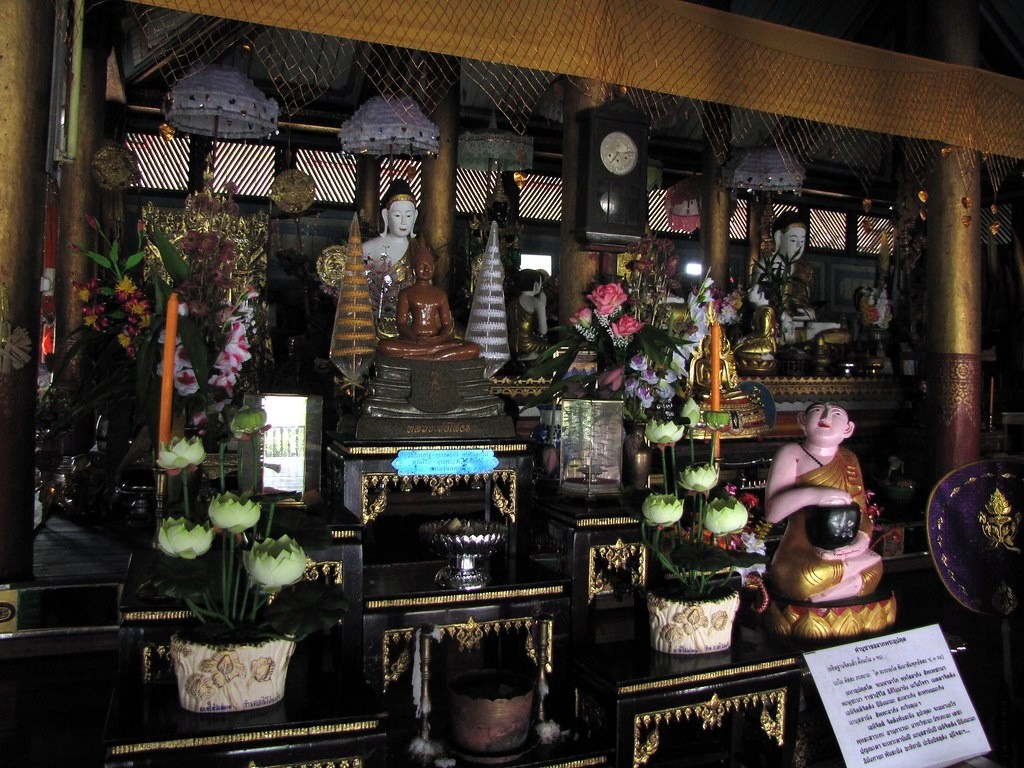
[171,627,296,715]
[143,396,174,455]
[621,421,654,493]
[645,590,742,656]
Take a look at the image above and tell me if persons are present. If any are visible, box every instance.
[765,400,883,600]
[746,210,893,344]
[362,179,564,361]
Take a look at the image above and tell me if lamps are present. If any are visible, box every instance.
[161,62,282,143]
[716,142,806,201]
[336,94,441,164]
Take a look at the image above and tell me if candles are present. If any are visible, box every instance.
[711,318,722,412]
[989,376,995,415]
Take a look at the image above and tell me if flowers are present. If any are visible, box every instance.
[857,188,930,331]
[634,398,772,604]
[134,403,352,643]
[508,231,750,432]
[62,139,272,454]
[745,235,815,344]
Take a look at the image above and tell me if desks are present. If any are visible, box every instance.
[563,598,967,768]
